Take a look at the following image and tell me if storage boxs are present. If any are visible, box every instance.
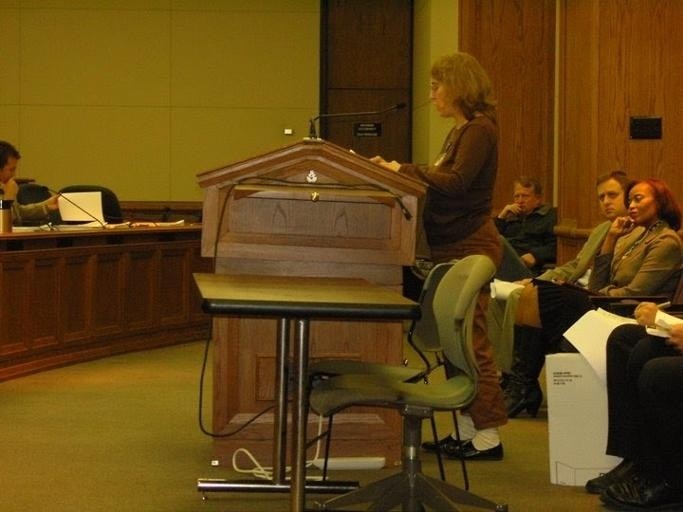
[546,353,623,489]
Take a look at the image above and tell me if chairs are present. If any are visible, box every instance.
[544,243,682,323]
[16,184,49,206]
[56,185,123,224]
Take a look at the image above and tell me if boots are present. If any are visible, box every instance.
[501,323,545,418]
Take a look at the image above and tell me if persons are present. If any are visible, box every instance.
[495,172,560,278]
[498,176,681,418]
[371,50,510,463]
[0,140,60,226]
[487,172,631,377]
[589,301,682,510]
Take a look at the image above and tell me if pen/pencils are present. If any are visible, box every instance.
[631,300,671,317]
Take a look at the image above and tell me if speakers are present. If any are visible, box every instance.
[630,117,661,139]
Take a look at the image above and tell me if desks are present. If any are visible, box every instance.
[192,273,422,511]
[0,221,212,383]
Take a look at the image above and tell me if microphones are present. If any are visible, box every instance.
[42,186,106,229]
[303,102,406,143]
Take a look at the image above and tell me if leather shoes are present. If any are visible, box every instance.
[422,433,468,453]
[448,439,504,461]
[602,470,682,511]
[585,463,633,493]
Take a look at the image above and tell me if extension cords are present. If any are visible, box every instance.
[314,457,385,471]
